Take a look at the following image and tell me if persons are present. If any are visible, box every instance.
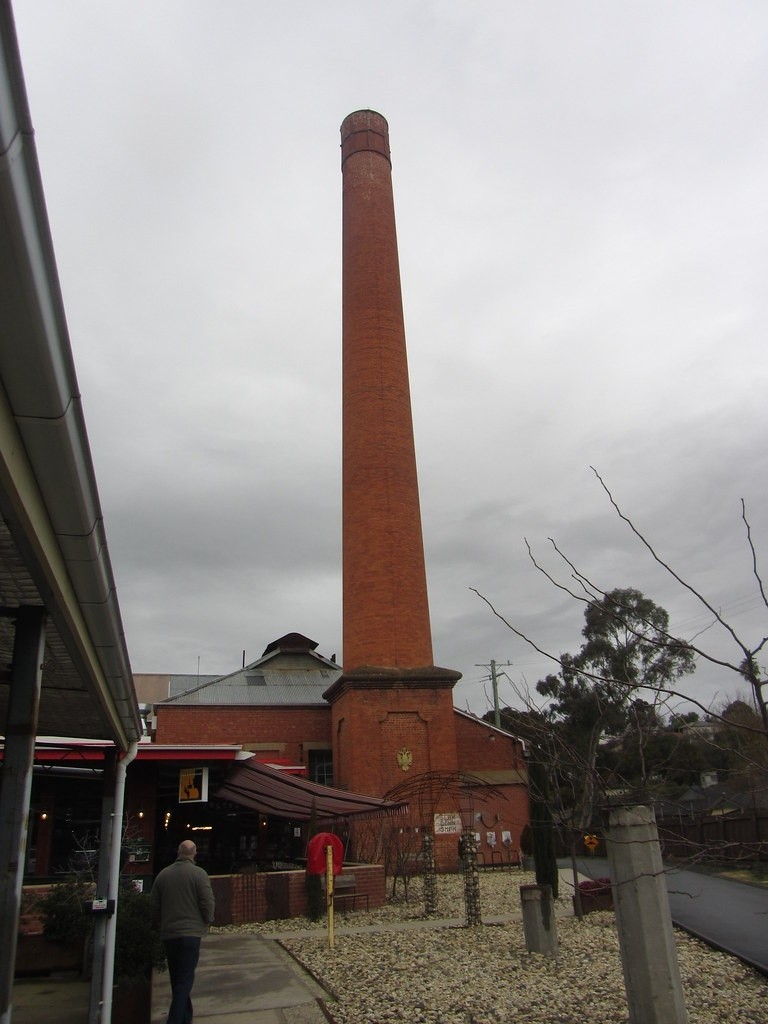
[149,838,215,1024]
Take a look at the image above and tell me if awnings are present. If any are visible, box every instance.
[212,757,409,830]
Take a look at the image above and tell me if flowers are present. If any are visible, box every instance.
[579,877,611,895]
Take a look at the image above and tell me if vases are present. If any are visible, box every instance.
[570,893,613,915]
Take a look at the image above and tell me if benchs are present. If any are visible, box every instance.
[320,874,369,912]
